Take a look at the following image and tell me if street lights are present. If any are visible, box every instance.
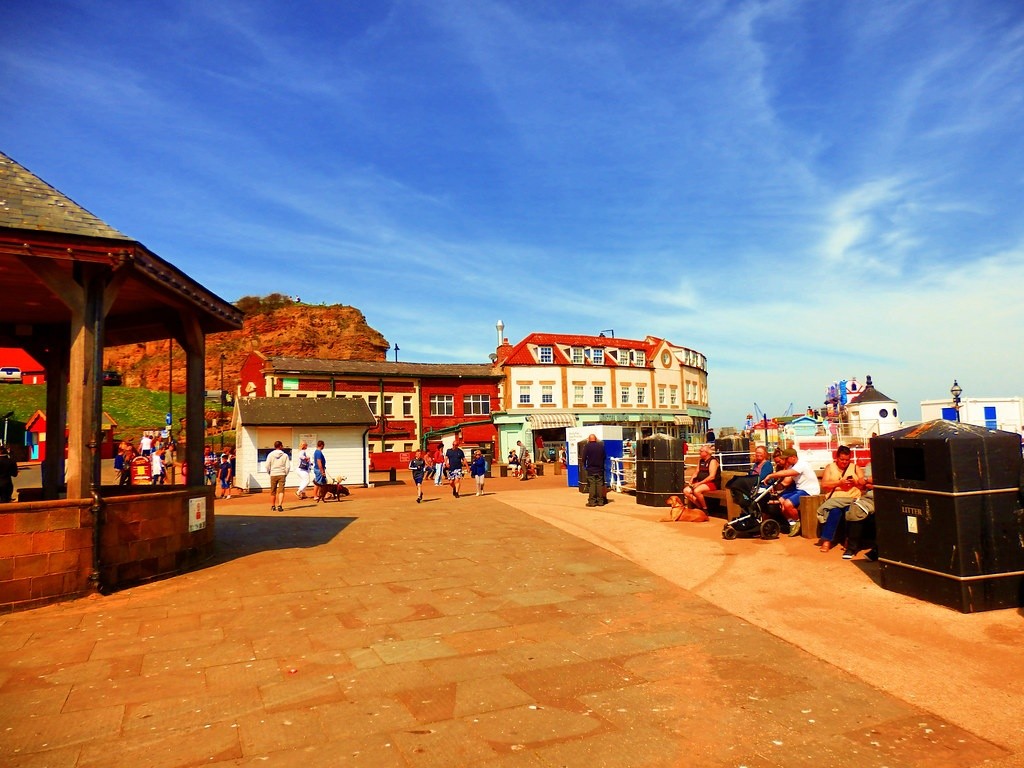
[218,353,228,450]
[949,379,963,422]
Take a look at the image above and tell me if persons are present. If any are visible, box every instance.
[293,441,313,499]
[64,458,68,493]
[842,463,879,561]
[265,440,290,511]
[408,438,490,503]
[683,445,722,516]
[507,434,568,482]
[582,434,608,506]
[806,405,820,419]
[312,440,327,501]
[706,427,715,444]
[725,444,821,537]
[112,415,237,501]
[0,442,19,504]
[819,445,864,551]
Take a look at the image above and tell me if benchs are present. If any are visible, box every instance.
[490,462,544,477]
[685,470,864,539]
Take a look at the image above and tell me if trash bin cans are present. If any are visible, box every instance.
[870,419,1024,614]
[714,434,750,472]
[471,454,491,477]
[635,434,685,508]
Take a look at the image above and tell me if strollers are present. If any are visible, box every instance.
[722,468,782,541]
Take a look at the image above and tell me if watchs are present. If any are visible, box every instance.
[863,476,868,483]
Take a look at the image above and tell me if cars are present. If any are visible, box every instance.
[0,367,26,384]
[102,370,122,387]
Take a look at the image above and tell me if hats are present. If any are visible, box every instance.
[476,450,481,454]
[517,441,520,445]
[781,448,797,459]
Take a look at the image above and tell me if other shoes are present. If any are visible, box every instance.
[417,498,420,503]
[547,459,551,462]
[314,497,319,500]
[295,492,302,500]
[843,538,849,550]
[842,549,855,559]
[226,495,231,499]
[455,492,459,498]
[445,477,448,479]
[453,489,456,495]
[476,493,480,496]
[272,506,275,510]
[586,504,594,507]
[820,540,830,551]
[598,504,603,506]
[481,490,484,494]
[278,506,283,511]
[863,549,879,561]
[430,477,434,480]
[789,519,800,536]
[426,476,428,480]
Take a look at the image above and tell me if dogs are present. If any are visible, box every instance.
[660,496,709,522]
[313,480,350,503]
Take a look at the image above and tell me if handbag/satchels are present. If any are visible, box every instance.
[299,459,309,470]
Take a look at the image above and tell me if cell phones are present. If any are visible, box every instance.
[847,476,852,480]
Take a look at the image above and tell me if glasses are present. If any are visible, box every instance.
[700,450,703,452]
